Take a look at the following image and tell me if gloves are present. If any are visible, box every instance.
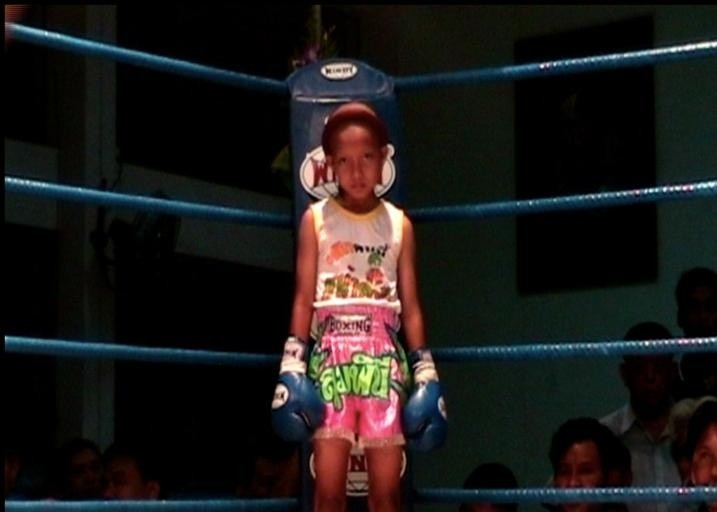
[404,347,450,455]
[271,334,323,445]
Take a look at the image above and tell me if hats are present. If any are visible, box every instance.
[322,102,387,155]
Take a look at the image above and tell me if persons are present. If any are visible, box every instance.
[2,429,303,499]
[269,102,449,512]
[457,266,716,511]
